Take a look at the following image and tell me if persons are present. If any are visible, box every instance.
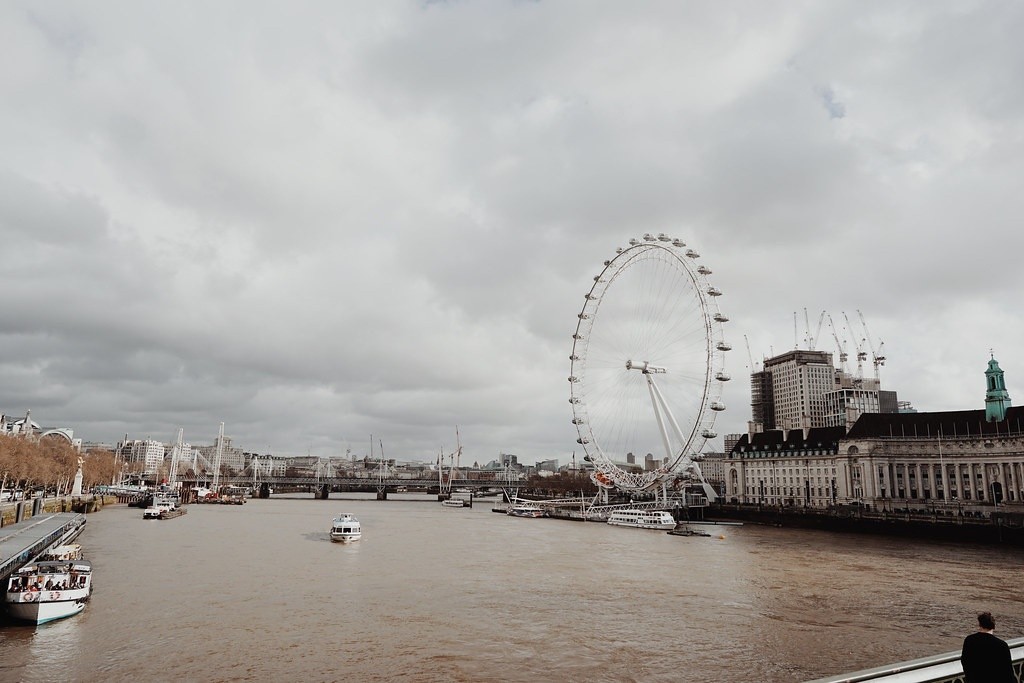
[961,612,1019,683]
[8,546,84,593]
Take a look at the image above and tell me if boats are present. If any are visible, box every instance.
[2,544,93,626]
[142,483,254,520]
[506,506,550,518]
[606,509,677,530]
[441,499,466,508]
[329,513,362,543]
